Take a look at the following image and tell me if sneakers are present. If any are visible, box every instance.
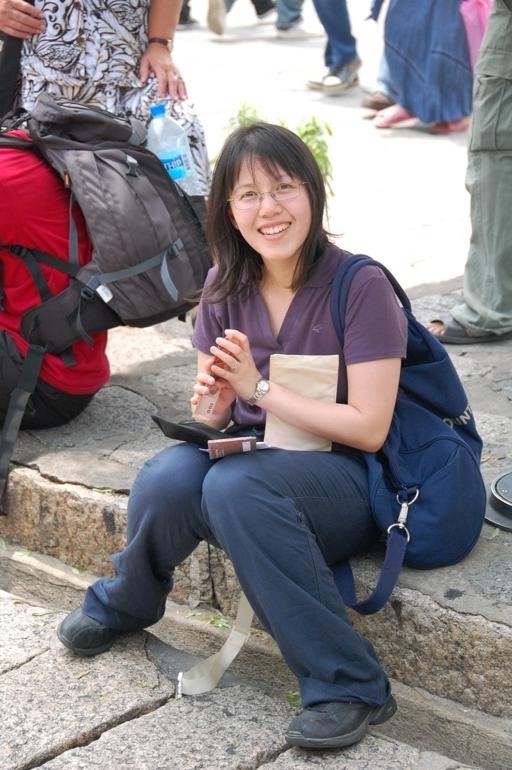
[57,604,119,655]
[176,0,323,41]
[306,54,470,134]
[285,692,397,749]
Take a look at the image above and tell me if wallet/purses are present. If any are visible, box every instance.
[152,416,231,445]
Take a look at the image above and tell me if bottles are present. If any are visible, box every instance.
[146,105,202,196]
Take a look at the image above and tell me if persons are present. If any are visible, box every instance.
[1,1,213,204]
[56,119,412,756]
[426,0,512,345]
[2,113,110,432]
[178,0,492,134]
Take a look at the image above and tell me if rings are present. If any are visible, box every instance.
[173,73,183,83]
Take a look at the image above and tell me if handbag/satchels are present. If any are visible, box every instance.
[331,255,485,570]
[0,32,22,117]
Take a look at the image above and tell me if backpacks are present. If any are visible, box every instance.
[1,93,211,353]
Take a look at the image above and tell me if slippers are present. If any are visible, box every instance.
[424,315,512,344]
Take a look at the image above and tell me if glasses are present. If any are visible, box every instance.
[221,178,306,210]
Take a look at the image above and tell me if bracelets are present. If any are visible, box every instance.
[148,36,174,53]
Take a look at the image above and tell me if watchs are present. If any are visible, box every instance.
[245,377,271,405]
